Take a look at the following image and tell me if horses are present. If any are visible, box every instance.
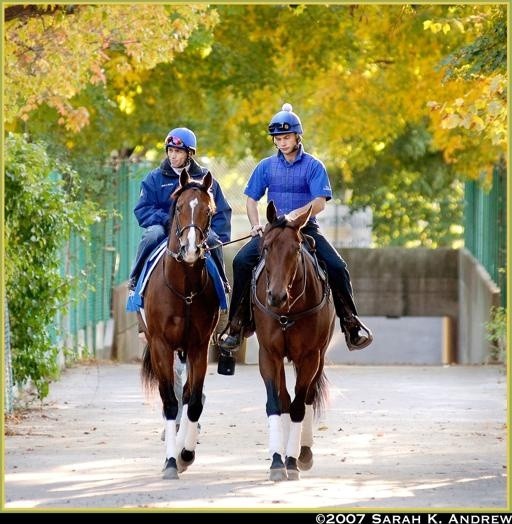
[250,200,337,483]
[135,167,222,482]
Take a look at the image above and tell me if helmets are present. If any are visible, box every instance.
[165,128,196,153]
[268,111,304,135]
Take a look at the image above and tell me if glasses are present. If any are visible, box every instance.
[166,136,189,148]
[268,123,300,131]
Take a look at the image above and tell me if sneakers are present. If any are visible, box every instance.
[347,316,370,347]
[221,330,244,348]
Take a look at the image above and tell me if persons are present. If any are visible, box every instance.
[219,102,368,350]
[126,127,232,347]
